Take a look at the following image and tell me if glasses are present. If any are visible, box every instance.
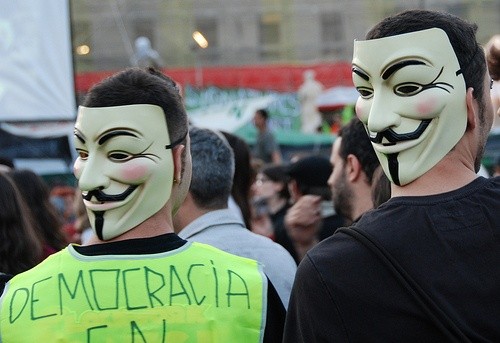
[255,177,272,187]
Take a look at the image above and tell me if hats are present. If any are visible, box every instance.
[285,157,333,184]
[263,166,291,182]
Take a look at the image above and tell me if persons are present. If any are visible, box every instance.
[0,36,500,311]
[0,66,286,342]
[284,10,500,343]
